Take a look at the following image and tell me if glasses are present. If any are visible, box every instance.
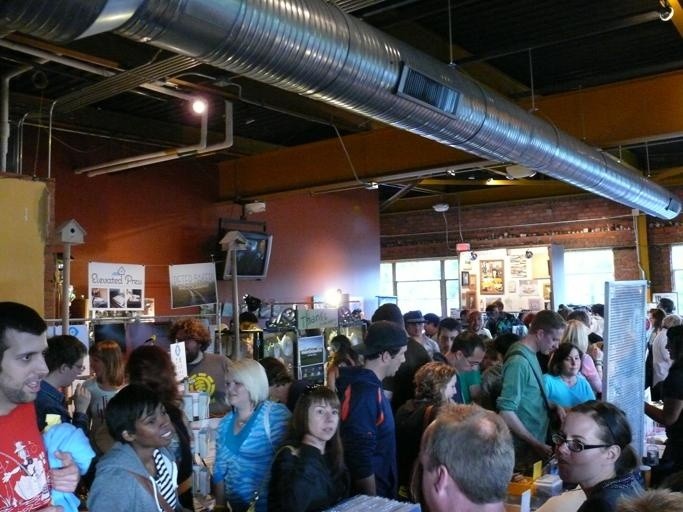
[553,433,608,452]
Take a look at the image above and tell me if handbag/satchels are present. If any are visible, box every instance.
[481,360,503,410]
[407,455,424,503]
[240,500,257,512]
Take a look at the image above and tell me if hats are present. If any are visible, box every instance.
[405,311,428,324]
[367,321,407,355]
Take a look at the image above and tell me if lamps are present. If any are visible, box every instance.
[658,0,674,22]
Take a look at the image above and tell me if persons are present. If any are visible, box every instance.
[0,296,292,512]
[269,385,340,510]
[262,359,313,414]
[327,297,682,511]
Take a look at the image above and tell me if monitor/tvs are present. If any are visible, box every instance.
[216,230,273,281]
